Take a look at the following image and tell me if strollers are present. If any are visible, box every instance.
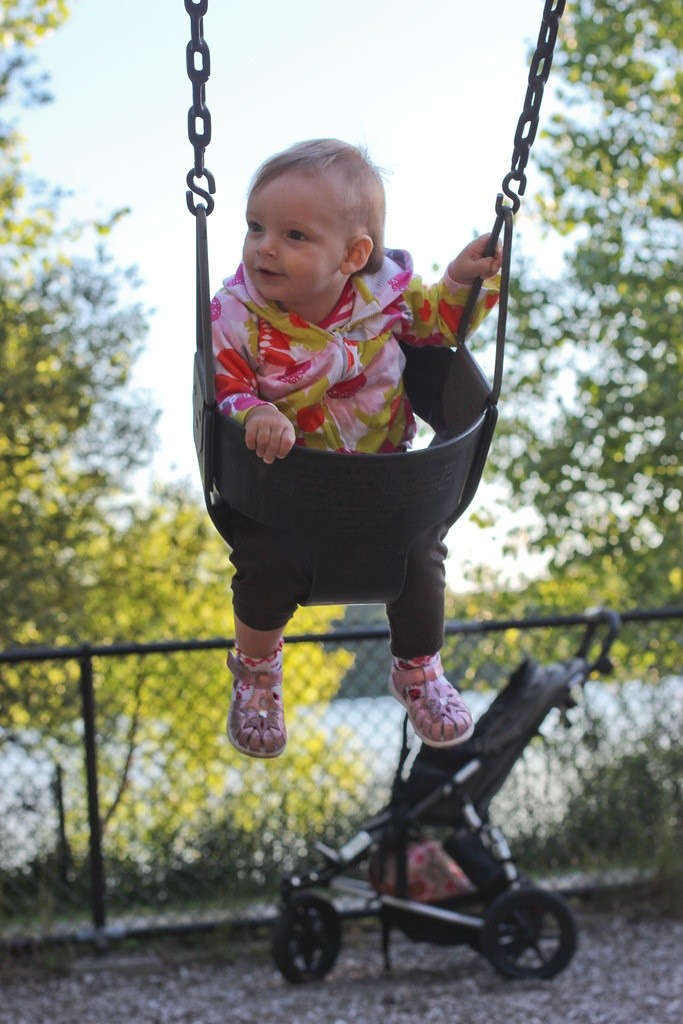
[270,611,623,991]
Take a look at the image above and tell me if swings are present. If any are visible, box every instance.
[181,0,569,608]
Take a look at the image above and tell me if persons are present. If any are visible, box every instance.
[199,138,507,759]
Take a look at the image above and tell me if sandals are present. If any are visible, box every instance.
[226,649,288,758]
[388,652,475,748]
